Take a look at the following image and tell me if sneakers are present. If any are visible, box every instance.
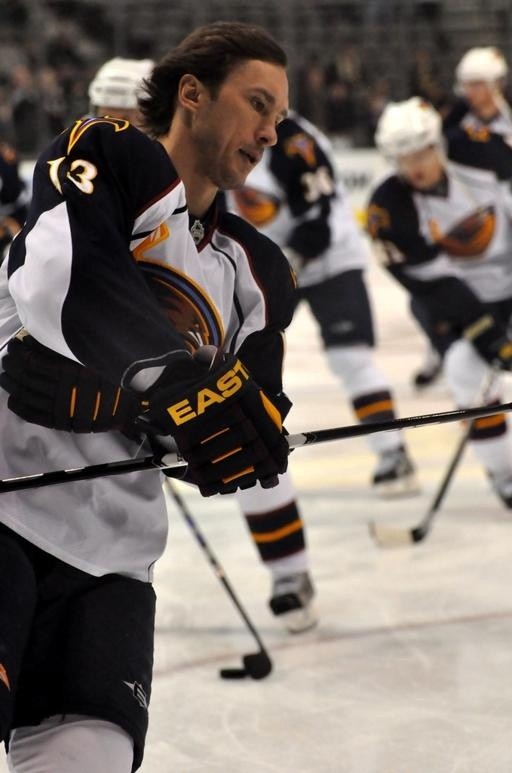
[371,443,418,484]
[266,568,318,616]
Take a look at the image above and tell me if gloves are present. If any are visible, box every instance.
[0,331,134,435]
[146,353,294,498]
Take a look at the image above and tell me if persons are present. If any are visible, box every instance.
[1,18,304,773]
[0,0,512,632]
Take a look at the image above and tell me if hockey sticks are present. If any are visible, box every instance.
[369,359,502,546]
[164,478,273,680]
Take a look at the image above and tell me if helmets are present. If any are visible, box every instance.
[372,95,444,158]
[86,53,158,112]
[451,45,509,84]
